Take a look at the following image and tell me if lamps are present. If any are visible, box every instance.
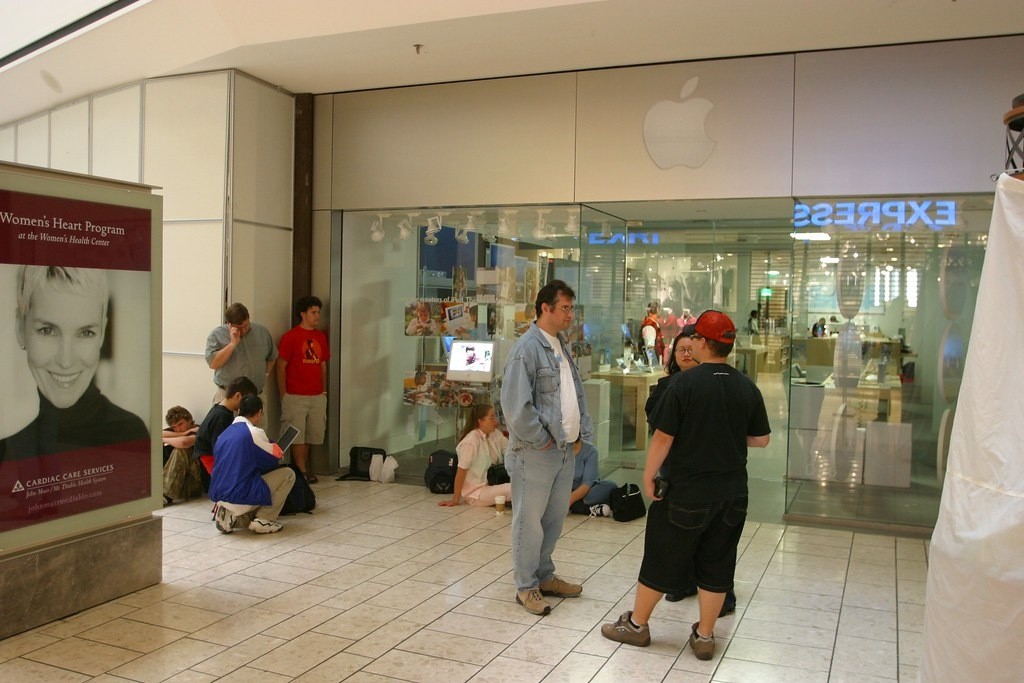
[373,212,612,246]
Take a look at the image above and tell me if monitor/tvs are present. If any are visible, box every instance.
[441,335,456,356]
[446,337,494,384]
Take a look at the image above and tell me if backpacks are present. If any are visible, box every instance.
[279,464,316,516]
[424,450,458,494]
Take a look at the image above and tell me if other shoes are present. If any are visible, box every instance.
[163,495,173,507]
[665,588,697,602]
[303,470,318,484]
[717,602,736,617]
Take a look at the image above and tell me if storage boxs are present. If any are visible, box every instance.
[790,386,840,431]
[594,419,609,460]
[582,380,609,423]
[864,421,913,487]
[789,428,866,484]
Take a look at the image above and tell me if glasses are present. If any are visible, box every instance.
[690,334,709,344]
[674,346,693,355]
[553,305,575,313]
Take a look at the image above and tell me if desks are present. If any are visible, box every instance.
[589,338,902,450]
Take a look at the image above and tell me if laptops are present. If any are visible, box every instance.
[276,424,300,453]
[791,372,833,387]
[445,303,469,329]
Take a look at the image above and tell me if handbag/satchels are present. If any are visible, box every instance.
[335,446,386,483]
[370,455,399,483]
[610,482,646,521]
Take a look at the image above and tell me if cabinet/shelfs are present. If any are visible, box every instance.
[404,332,501,459]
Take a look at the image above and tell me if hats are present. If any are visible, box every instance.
[694,310,736,344]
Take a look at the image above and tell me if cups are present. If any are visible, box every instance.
[495,496,506,516]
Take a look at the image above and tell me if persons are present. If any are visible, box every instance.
[162,406,205,505]
[437,405,513,508]
[194,377,258,494]
[812,317,826,338]
[645,330,740,614]
[659,308,678,344]
[570,430,619,517]
[676,309,698,335]
[748,310,759,335]
[204,301,277,431]
[207,394,297,533]
[828,316,840,336]
[0,263,149,459]
[276,295,331,485]
[600,308,771,662]
[501,277,595,617]
[637,301,665,363]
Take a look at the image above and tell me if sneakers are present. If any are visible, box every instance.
[516,590,550,615]
[216,505,236,534]
[690,622,714,660]
[248,518,283,533]
[601,611,650,647]
[589,504,611,518]
[539,574,582,598]
